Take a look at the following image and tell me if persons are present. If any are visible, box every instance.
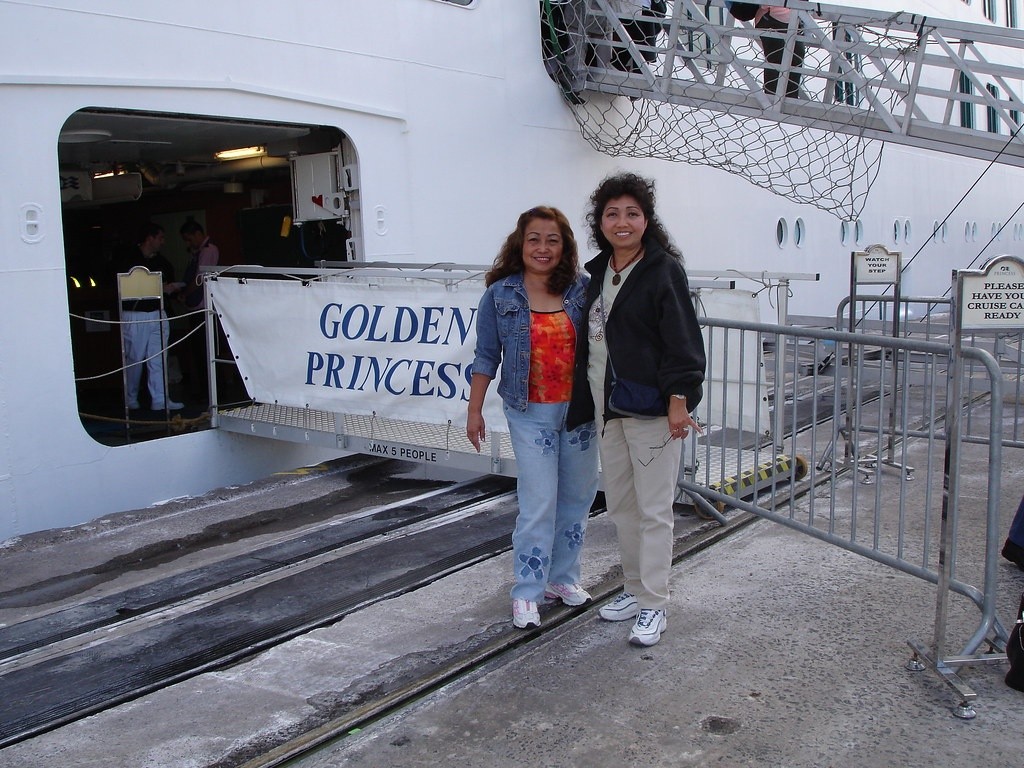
[755,0,809,99]
[113,224,184,410]
[164,220,230,407]
[610,0,667,74]
[466,206,599,629]
[565,171,706,646]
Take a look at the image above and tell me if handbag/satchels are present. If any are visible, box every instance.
[607,377,667,420]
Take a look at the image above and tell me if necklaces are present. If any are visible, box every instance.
[608,246,642,286]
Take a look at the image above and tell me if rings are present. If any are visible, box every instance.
[684,428,689,431]
[674,429,678,432]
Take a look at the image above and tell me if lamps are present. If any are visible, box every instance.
[211,146,269,162]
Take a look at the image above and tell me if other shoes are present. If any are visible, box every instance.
[151,398,185,411]
[128,400,139,410]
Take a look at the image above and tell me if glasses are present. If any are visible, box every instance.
[638,431,674,467]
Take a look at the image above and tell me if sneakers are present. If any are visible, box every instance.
[512,598,541,629]
[598,593,640,621]
[544,582,592,606]
[629,608,667,646]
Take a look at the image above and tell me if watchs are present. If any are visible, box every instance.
[673,395,686,400]
